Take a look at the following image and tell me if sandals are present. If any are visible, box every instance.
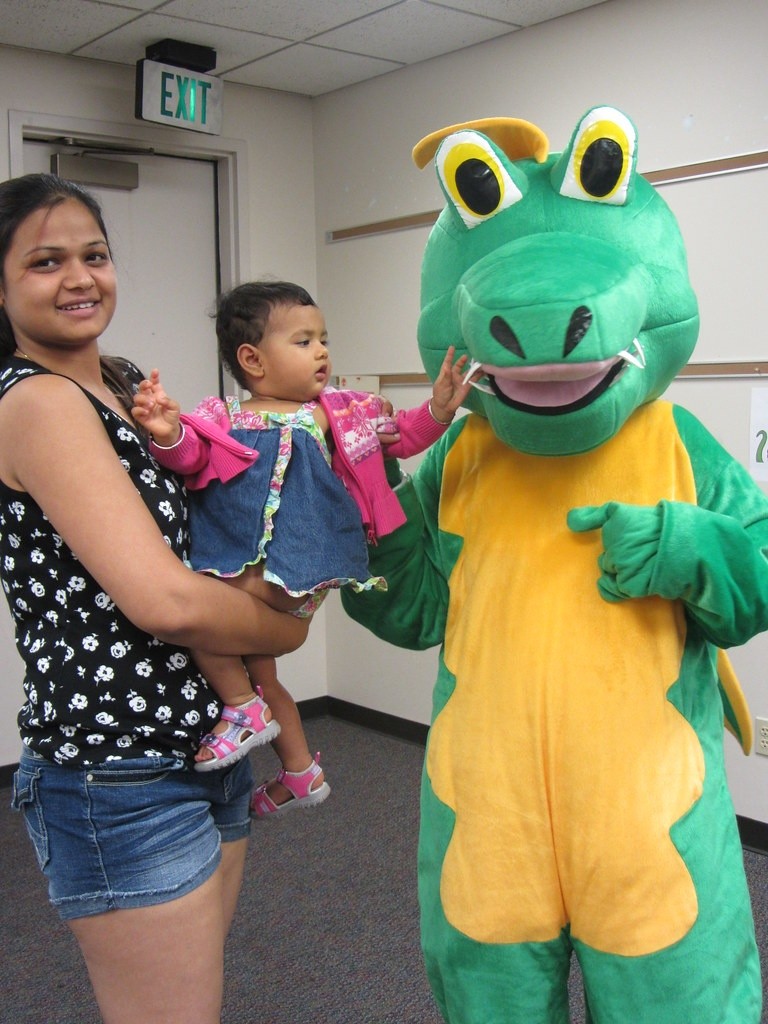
[194,686,279,773]
[249,751,331,820]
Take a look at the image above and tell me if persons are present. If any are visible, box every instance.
[0,174,311,1024]
[131,281,482,822]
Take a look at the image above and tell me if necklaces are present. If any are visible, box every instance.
[15,349,33,362]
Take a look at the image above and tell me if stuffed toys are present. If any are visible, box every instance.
[341,101,767,1024]
